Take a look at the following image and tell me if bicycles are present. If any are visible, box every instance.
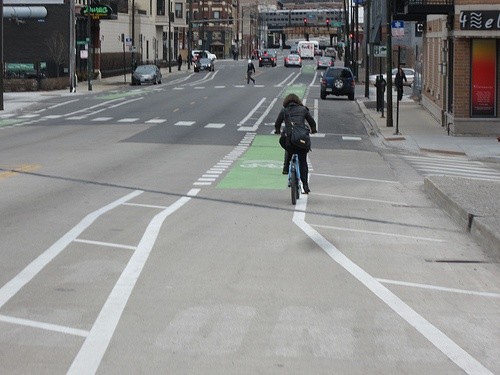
[276,129,313,205]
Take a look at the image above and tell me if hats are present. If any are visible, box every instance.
[248,59,251,63]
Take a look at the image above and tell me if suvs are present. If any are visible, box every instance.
[192,50,216,61]
[320,66,357,101]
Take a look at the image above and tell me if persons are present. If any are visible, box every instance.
[232,47,240,62]
[251,48,258,60]
[247,59,255,84]
[178,54,182,70]
[395,68,408,101]
[274,93,316,194]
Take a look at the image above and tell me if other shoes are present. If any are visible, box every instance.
[247,82,249,84]
[304,186,310,193]
[253,80,255,84]
[283,165,289,172]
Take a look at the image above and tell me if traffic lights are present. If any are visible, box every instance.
[304,18,307,25]
[326,17,329,26]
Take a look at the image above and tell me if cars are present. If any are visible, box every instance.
[132,65,163,85]
[194,58,214,72]
[259,41,337,68]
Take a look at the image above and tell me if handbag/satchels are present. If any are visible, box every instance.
[394,85,397,91]
[291,125,311,150]
